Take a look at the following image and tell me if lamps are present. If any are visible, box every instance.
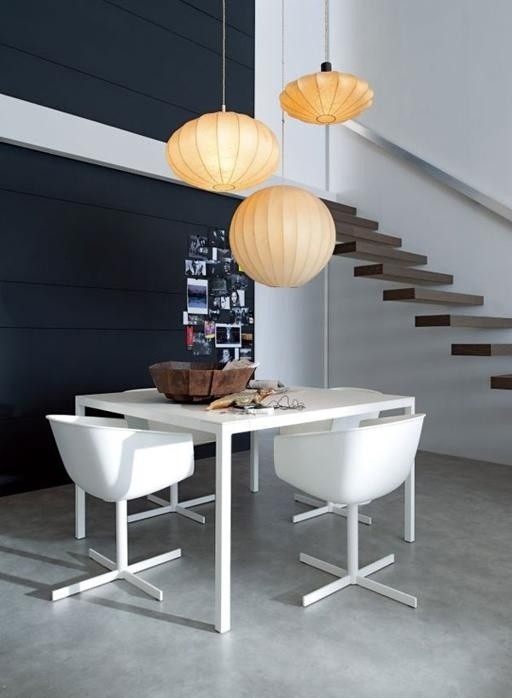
[278,1,373,123]
[165,0,281,193]
[227,0,337,290]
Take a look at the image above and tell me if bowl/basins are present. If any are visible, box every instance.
[148,359,258,404]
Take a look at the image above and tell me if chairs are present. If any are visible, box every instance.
[273,413,428,609]
[292,387,381,524]
[45,414,195,603]
[125,388,214,524]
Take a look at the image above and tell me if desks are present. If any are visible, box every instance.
[75,388,416,634]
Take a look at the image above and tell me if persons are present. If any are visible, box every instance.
[188,229,253,363]
